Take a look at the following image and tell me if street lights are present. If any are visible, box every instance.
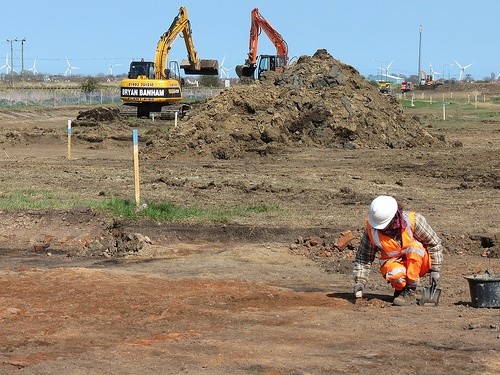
[7,38,16,75]
[14,37,25,74]
[416,25,424,87]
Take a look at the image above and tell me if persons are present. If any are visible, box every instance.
[353,196,444,305]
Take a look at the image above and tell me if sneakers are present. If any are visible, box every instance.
[393,288,417,306]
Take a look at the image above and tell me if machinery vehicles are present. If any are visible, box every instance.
[234,7,293,83]
[400,82,413,93]
[120,4,221,119]
[377,80,391,94]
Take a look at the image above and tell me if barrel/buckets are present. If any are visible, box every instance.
[465,275,500,308]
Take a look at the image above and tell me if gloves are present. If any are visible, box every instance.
[353,282,364,297]
[429,271,440,286]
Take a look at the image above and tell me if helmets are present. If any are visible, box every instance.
[368,196,398,230]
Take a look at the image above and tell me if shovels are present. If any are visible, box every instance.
[418,281,442,308]
